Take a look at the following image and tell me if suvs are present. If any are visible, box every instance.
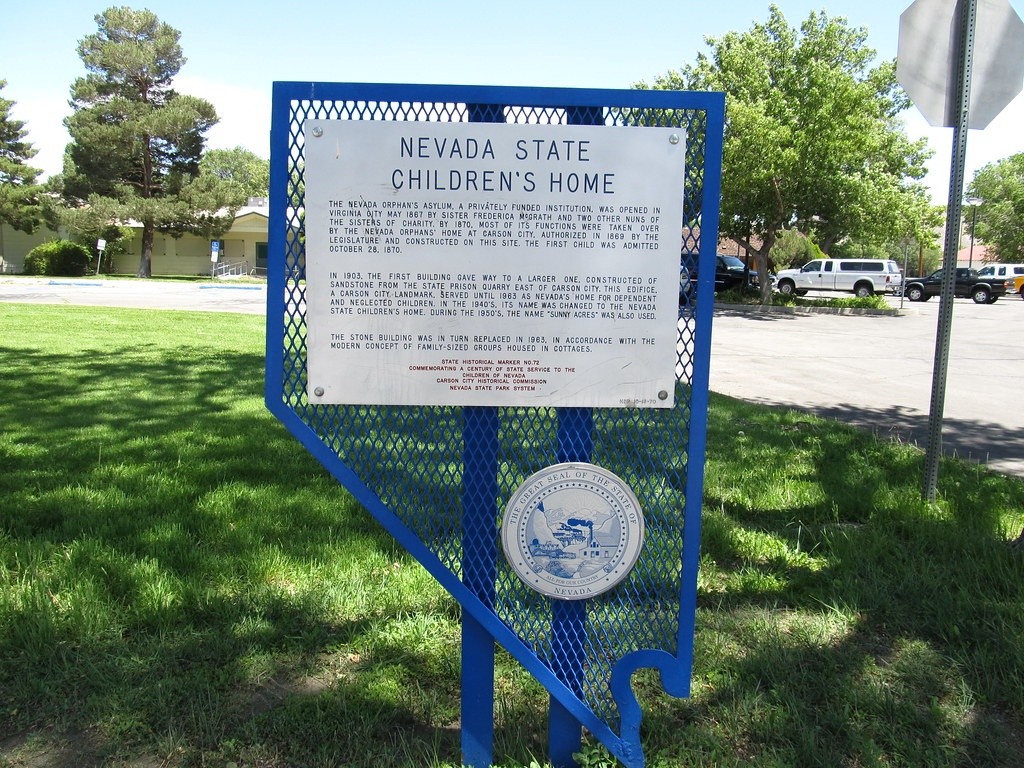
[776,258,901,297]
[681,252,775,289]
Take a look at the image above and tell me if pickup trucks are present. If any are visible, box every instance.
[905,267,1008,304]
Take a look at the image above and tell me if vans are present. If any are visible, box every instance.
[976,264,1024,279]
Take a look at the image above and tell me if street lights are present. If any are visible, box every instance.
[968,196,983,267]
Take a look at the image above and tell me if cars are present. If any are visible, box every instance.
[1004,276,1024,300]
